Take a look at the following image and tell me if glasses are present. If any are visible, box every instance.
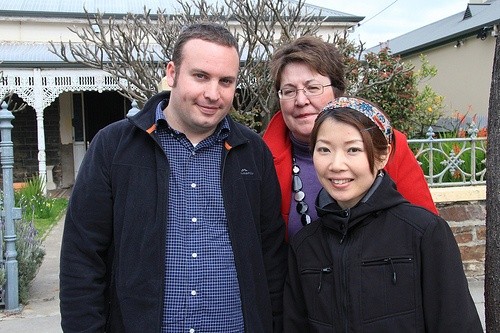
[278,84,332,100]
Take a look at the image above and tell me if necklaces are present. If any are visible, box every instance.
[292,157,313,226]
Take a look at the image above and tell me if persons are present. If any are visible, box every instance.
[282,95,483,333]
[263,38,439,239]
[59,21,297,332]
